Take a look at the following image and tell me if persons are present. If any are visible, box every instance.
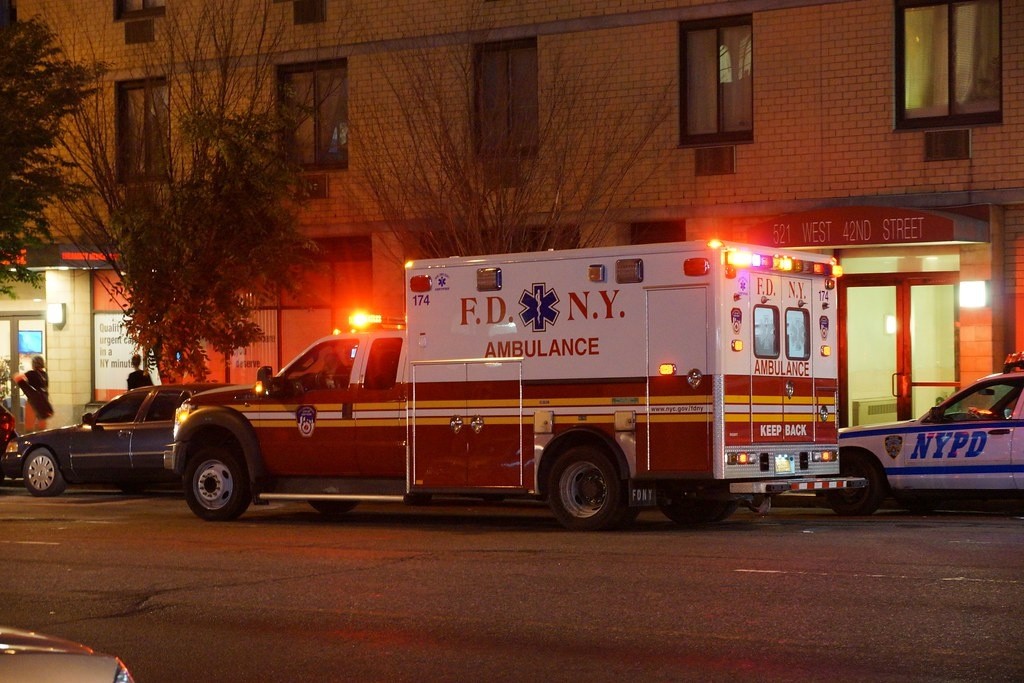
[14,355,49,433]
[127,354,153,390]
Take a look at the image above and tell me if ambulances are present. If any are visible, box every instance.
[166,238,869,532]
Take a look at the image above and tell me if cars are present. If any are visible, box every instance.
[1,380,238,497]
[814,349,1024,516]
